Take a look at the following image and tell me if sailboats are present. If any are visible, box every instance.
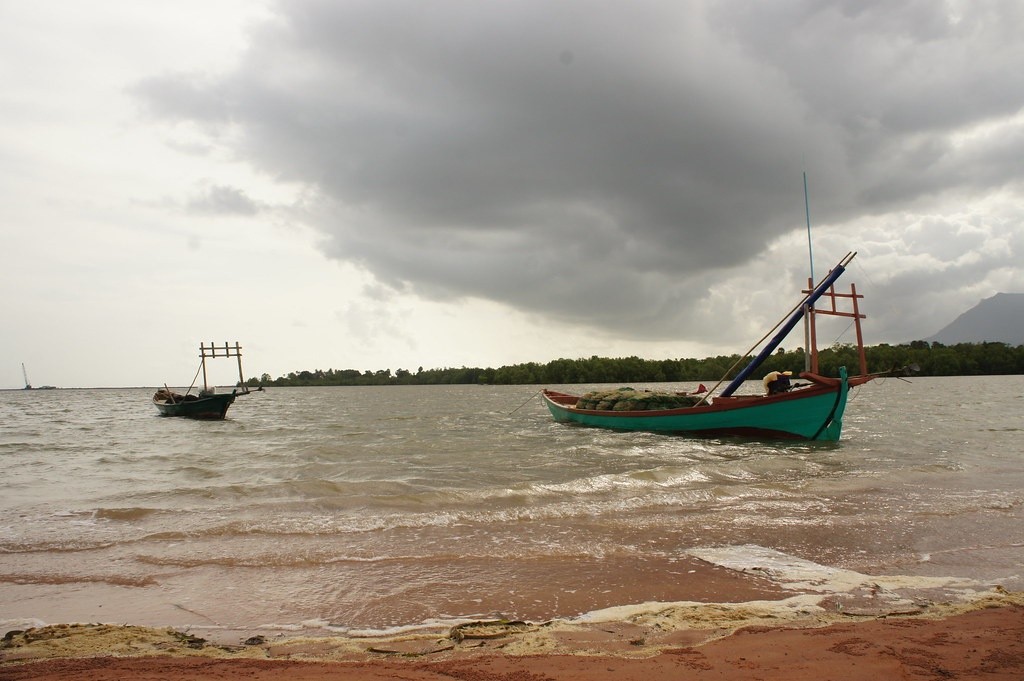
[20,363,56,390]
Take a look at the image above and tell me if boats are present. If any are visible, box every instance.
[540,172,920,443]
[149,342,266,421]
